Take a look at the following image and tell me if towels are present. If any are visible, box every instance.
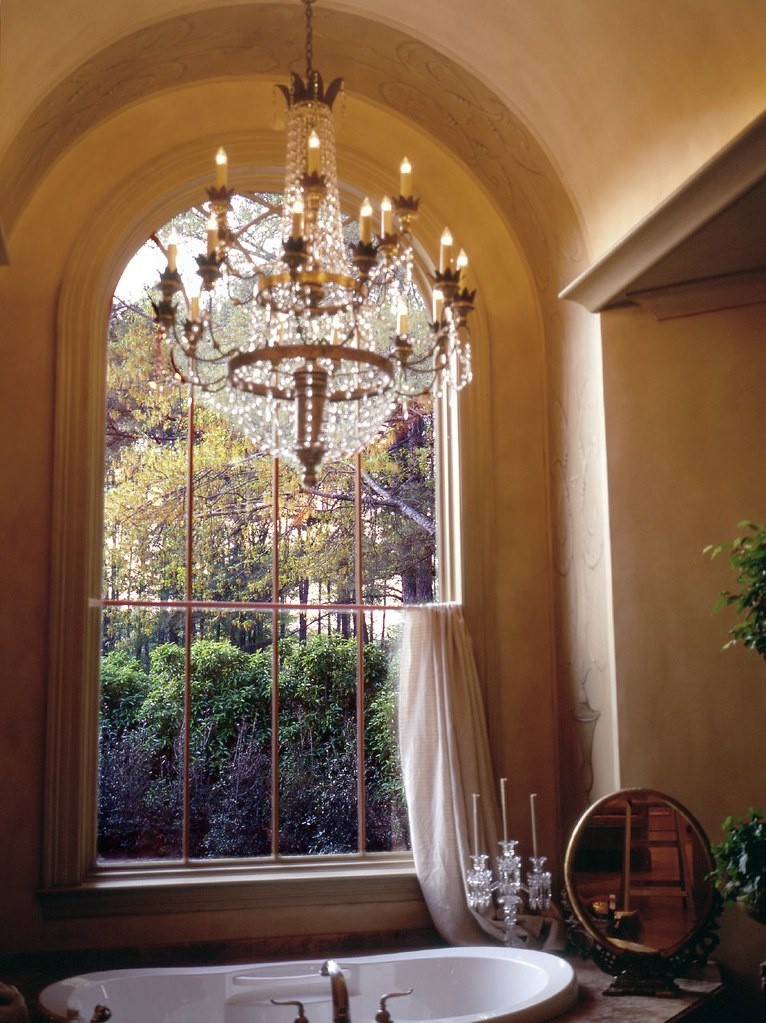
[0,982,29,1023]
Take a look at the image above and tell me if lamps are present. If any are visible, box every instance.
[466,774,551,947]
[151,0,477,490]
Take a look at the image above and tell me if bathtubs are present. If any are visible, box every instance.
[40,948,577,1022]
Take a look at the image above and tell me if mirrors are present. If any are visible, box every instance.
[561,787,726,999]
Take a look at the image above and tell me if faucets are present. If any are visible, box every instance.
[321,960,351,1021]
